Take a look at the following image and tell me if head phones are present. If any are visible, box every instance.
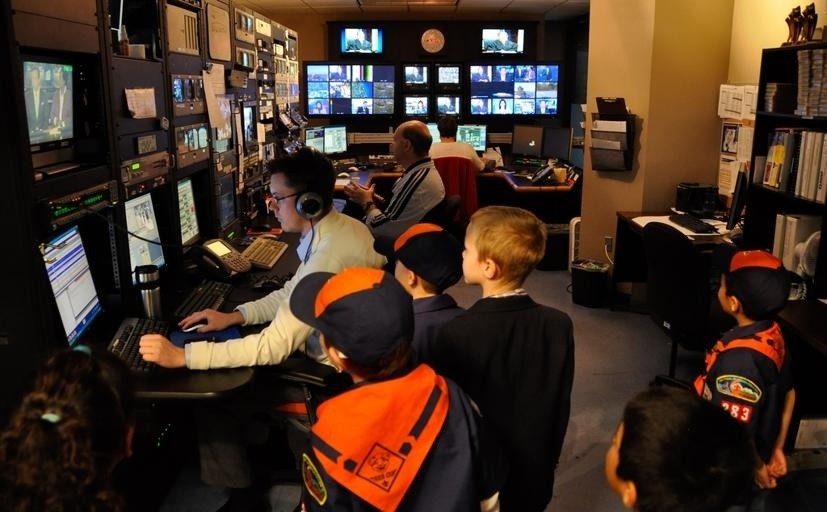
[296,146,324,219]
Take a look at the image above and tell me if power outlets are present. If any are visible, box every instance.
[603,236,614,253]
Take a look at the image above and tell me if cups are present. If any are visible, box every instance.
[553,167,566,184]
[136,264,163,322]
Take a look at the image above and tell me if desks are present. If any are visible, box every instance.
[611,212,742,338]
[325,154,579,198]
[117,195,353,400]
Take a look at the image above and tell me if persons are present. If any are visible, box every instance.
[347,32,372,52]
[484,31,518,51]
[48,68,72,129]
[373,223,467,366]
[430,118,489,172]
[312,66,553,114]
[138,146,388,512]
[431,206,575,512]
[25,67,50,134]
[606,391,751,512]
[694,250,796,512]
[0,346,140,512]
[344,119,446,276]
[289,266,501,512]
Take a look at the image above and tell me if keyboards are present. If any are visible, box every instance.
[670,213,718,234]
[172,279,235,323]
[335,162,356,174]
[241,238,288,270]
[107,317,169,380]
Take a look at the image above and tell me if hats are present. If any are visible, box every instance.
[373,222,463,286]
[288,264,415,365]
[713,243,792,319]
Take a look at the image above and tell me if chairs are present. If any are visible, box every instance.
[425,192,466,227]
[432,155,477,211]
[257,368,333,450]
[638,220,740,391]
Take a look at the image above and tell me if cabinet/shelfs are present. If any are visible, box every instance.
[746,37,827,356]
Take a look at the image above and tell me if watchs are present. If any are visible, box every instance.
[360,201,375,211]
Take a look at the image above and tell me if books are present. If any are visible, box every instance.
[763,127,827,272]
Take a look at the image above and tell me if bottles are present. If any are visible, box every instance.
[117,24,129,55]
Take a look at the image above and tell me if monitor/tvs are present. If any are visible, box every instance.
[726,162,748,246]
[302,20,560,117]
[177,176,201,253]
[124,192,166,285]
[422,122,440,144]
[20,53,94,182]
[171,14,299,157]
[511,124,545,159]
[39,223,103,348]
[454,125,487,153]
[305,126,323,155]
[322,125,349,158]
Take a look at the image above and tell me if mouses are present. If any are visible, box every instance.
[181,319,207,333]
[338,172,350,178]
[349,167,359,171]
[252,223,272,231]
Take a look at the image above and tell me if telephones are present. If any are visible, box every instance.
[530,164,555,185]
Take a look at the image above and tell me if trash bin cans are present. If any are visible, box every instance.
[568,258,611,310]
